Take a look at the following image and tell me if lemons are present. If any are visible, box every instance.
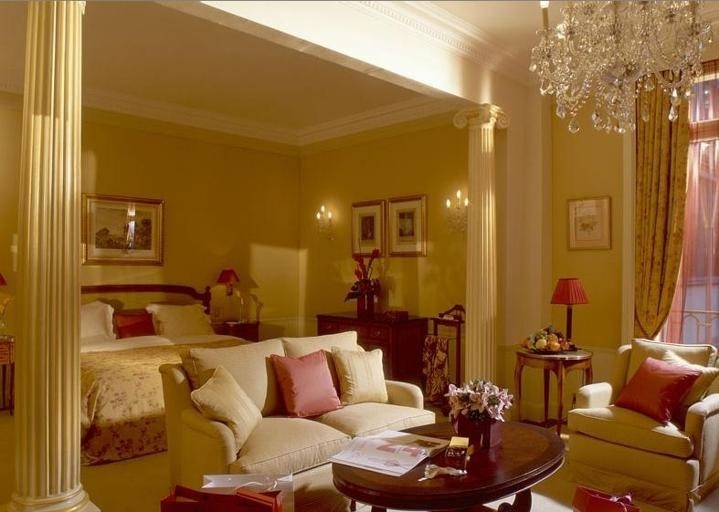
[551,342,561,351]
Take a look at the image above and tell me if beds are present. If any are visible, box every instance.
[80,282,258,469]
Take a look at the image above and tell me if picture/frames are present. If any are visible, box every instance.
[385,191,432,259]
[350,196,389,259]
[564,194,617,253]
[77,193,168,266]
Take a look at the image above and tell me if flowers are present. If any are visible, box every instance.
[442,376,517,430]
[341,249,390,301]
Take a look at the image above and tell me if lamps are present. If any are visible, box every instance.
[440,187,474,230]
[548,275,594,348]
[215,268,251,330]
[313,202,339,243]
[526,0,717,136]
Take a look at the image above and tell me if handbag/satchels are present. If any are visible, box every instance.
[573,486,639,512]
[161,473,294,512]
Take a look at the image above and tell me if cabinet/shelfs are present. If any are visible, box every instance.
[313,309,433,394]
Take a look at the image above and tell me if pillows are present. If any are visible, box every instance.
[660,347,719,425]
[268,349,344,423]
[329,343,390,406]
[186,361,265,461]
[113,306,159,342]
[610,354,702,428]
[145,301,216,339]
[78,296,118,344]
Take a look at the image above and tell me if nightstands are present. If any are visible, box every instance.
[211,316,263,345]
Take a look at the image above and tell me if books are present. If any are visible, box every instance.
[331,427,451,477]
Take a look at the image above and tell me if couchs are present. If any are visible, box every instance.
[158,327,439,511]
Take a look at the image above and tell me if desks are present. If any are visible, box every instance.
[330,416,566,512]
[507,344,597,444]
[0,334,20,421]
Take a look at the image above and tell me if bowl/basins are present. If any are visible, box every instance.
[384,309,409,322]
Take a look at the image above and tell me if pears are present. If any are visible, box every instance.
[536,339,546,349]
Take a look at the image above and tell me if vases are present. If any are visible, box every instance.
[456,413,504,451]
[354,291,379,317]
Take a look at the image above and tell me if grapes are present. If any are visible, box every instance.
[534,329,548,340]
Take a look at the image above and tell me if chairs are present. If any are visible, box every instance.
[564,338,719,512]
